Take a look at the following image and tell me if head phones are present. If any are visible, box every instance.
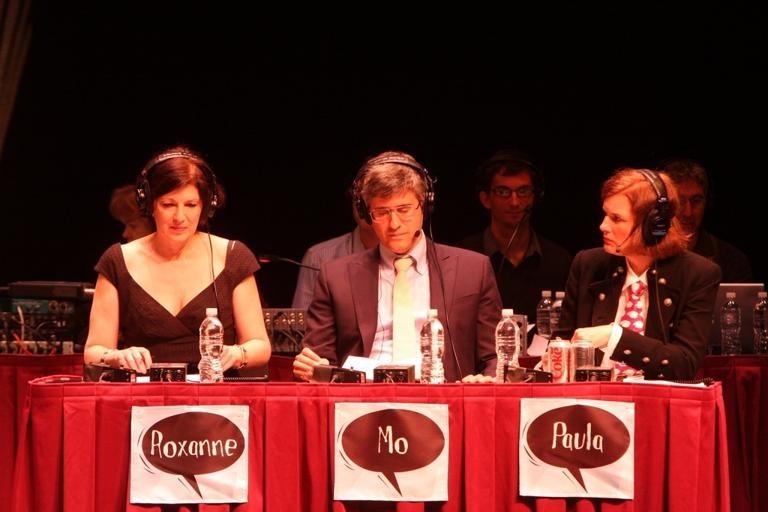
[634,170,674,248]
[136,153,218,218]
[354,159,435,225]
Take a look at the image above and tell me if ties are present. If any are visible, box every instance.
[393,258,416,365]
[615,281,644,376]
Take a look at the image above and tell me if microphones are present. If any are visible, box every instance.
[498,202,537,295]
[616,215,643,253]
[415,227,420,238]
[259,256,322,272]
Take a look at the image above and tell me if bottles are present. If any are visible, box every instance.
[550,292,565,334]
[548,340,571,384]
[420,309,444,384]
[198,308,224,383]
[536,290,553,338]
[495,308,520,384]
[569,340,595,383]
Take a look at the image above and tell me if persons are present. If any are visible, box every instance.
[552,166,721,382]
[293,164,381,309]
[655,155,750,283]
[83,146,272,374]
[462,153,573,318]
[111,184,157,243]
[292,151,505,384]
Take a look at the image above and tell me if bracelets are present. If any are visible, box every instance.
[234,343,248,370]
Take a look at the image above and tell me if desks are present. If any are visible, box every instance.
[0,348,768,512]
[14,375,727,512]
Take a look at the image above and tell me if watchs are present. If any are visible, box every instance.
[101,348,115,365]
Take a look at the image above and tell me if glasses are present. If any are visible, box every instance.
[367,200,420,223]
[487,184,532,198]
